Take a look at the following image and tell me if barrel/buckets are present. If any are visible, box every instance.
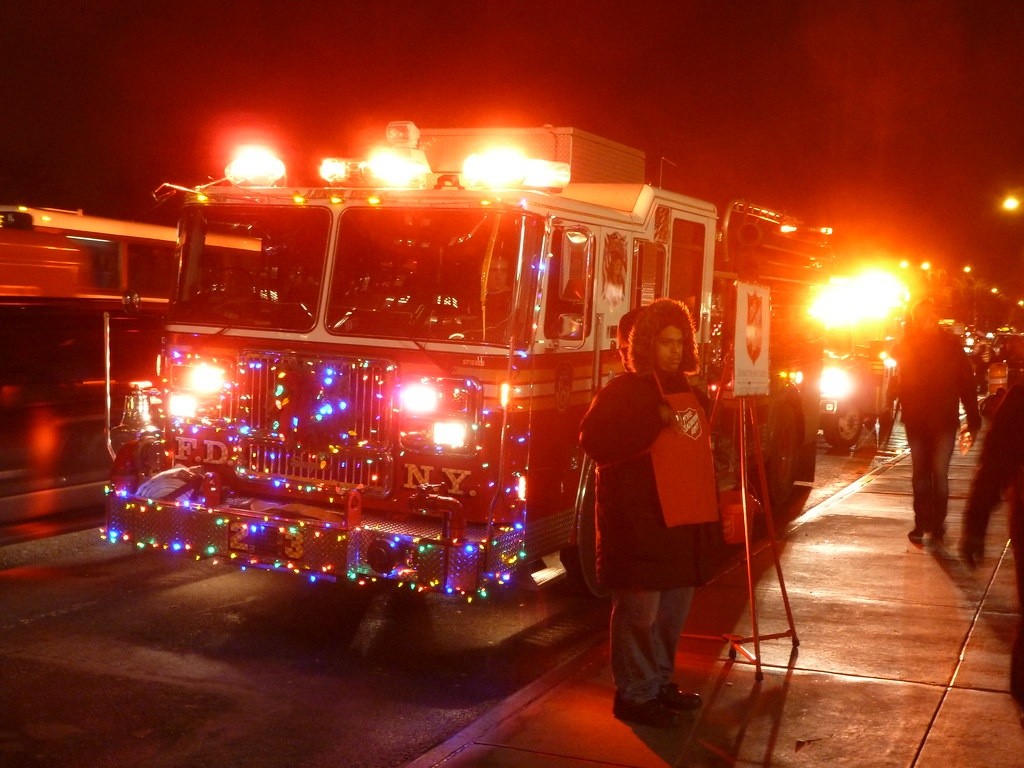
[717,491,755,544]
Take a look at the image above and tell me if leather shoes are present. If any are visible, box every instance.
[910,519,946,548]
[615,685,703,730]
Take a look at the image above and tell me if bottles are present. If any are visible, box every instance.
[959,423,972,455]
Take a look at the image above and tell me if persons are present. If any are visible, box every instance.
[970,331,1009,395]
[579,299,726,723]
[959,380,1024,699]
[890,299,983,543]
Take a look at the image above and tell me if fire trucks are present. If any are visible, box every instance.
[1,205,260,527]
[823,241,929,450]
[102,121,829,597]
